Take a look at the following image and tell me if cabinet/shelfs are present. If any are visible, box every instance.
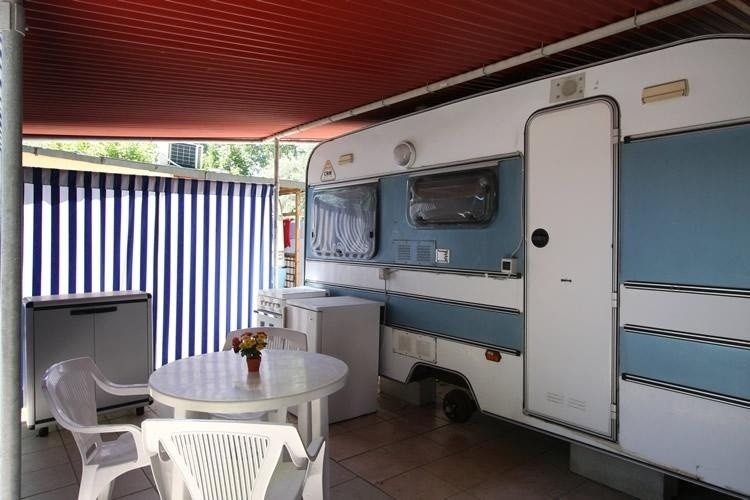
[23,289,156,438]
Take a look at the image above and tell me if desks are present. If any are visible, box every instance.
[139,347,353,500]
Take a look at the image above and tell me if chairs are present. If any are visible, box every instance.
[38,351,158,499]
[137,413,331,500]
[206,328,312,437]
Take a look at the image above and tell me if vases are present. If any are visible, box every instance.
[245,353,263,372]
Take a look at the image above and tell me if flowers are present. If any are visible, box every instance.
[231,328,269,355]
